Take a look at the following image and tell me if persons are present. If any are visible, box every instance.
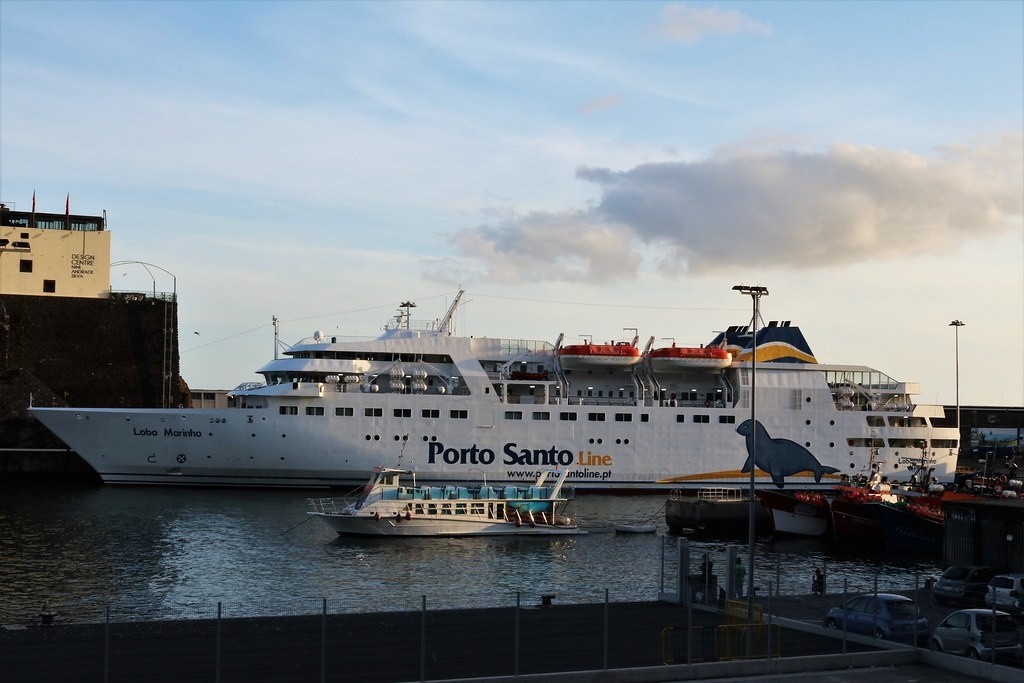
[839,462,945,494]
[733,558,747,599]
[812,569,824,597]
[699,553,713,574]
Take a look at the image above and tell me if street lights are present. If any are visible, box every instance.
[948,319,965,447]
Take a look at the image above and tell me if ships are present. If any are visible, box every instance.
[23,280,959,495]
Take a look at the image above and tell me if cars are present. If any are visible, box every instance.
[985,573,1024,616]
[823,592,929,641]
[934,566,1000,609]
[927,608,1024,662]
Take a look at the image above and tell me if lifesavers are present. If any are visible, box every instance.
[704,399,711,407]
[999,475,1007,482]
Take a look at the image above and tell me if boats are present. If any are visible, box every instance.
[755,428,943,560]
[558,344,640,373]
[307,433,589,538]
[650,347,734,374]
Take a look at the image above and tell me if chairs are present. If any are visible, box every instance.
[421,485,561,521]
